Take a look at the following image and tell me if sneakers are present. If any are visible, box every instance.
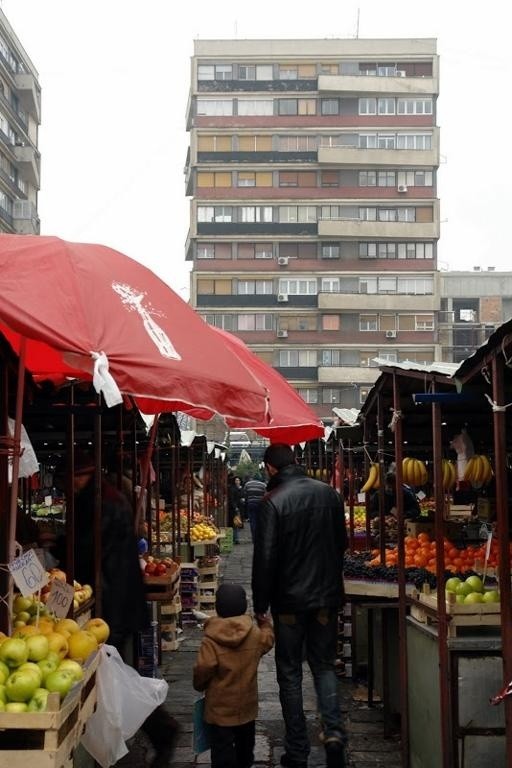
[322,738,347,767]
[279,752,309,768]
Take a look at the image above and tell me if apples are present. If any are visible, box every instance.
[446,575,499,604]
[0,568,110,712]
[143,562,168,578]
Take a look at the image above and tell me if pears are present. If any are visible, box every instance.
[186,523,217,543]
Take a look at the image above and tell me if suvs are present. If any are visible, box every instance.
[222,430,271,449]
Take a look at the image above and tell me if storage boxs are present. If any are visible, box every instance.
[343,581,416,604]
[477,495,496,522]
[407,521,460,546]
[161,533,226,563]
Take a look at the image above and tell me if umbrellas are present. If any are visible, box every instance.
[0,233,272,636]
[133,325,326,538]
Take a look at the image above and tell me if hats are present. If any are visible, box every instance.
[214,581,247,615]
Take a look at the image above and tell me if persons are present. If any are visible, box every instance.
[250,442,350,768]
[106,456,133,503]
[41,447,183,767]
[243,472,268,543]
[227,476,246,545]
[192,582,275,768]
[159,480,181,513]
[368,471,422,522]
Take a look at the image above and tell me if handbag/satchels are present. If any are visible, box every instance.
[233,514,242,527]
[190,690,211,755]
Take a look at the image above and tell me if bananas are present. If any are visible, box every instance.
[402,456,428,488]
[441,458,457,492]
[462,455,493,489]
[360,462,377,494]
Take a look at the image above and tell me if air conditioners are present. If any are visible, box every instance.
[277,330,288,338]
[397,184,408,193]
[279,257,288,265]
[386,330,397,338]
[277,293,288,303]
[394,71,406,77]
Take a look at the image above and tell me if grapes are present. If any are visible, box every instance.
[343,552,499,588]
